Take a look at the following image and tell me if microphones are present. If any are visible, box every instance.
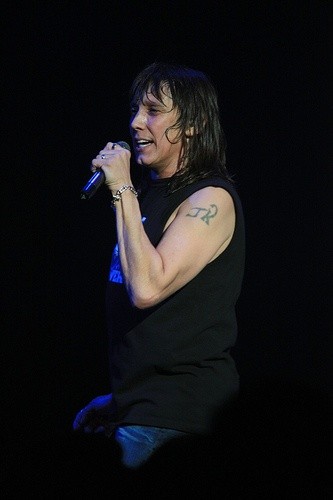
[81,140,130,200]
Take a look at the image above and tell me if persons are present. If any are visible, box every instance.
[74,60,245,500]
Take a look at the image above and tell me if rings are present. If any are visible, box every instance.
[111,143,117,150]
[80,407,89,415]
[101,154,106,159]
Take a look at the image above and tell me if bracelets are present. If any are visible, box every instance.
[110,184,138,210]
